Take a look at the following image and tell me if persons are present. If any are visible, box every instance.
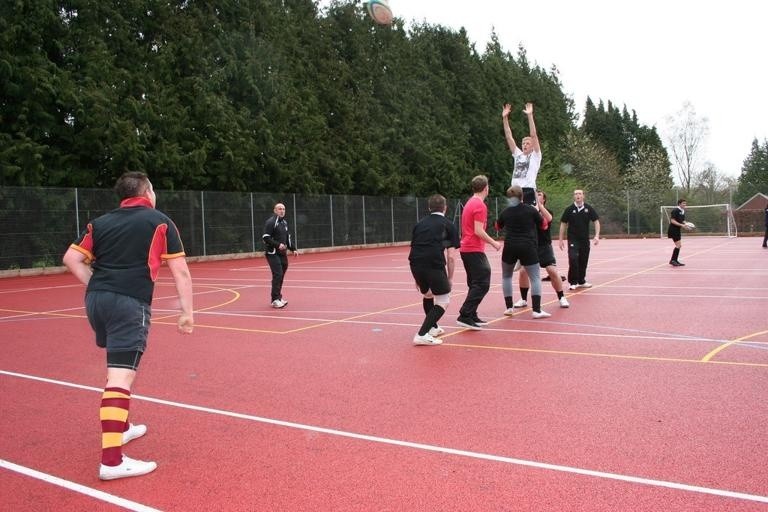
[408,194,461,345]
[514,190,570,307]
[762,203,768,247]
[456,175,501,331]
[495,186,551,319]
[502,102,542,271]
[63,171,196,482]
[668,199,689,267]
[558,190,601,290]
[262,203,299,309]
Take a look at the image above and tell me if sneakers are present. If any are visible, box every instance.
[428,323,445,337]
[669,259,685,266]
[455,313,489,330]
[99,453,159,481]
[504,308,513,316]
[413,333,443,346]
[121,423,147,446]
[531,309,551,319]
[558,296,569,308]
[514,298,528,308]
[568,283,592,290]
[272,299,287,309]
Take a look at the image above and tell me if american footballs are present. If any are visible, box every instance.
[367,0,393,25]
[685,223,696,231]
